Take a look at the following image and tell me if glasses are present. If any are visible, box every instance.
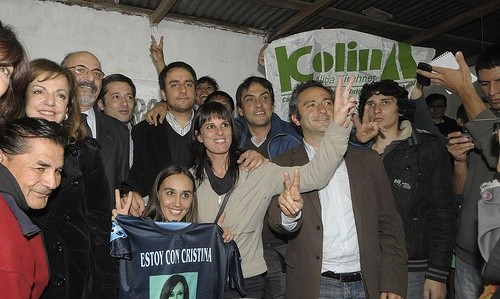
[67,63,105,79]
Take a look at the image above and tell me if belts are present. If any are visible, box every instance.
[321,268,363,283]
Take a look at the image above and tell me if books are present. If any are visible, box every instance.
[424,52,478,94]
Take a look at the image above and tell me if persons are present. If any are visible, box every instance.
[268,79,407,299]
[416,51,500,299]
[110,166,247,299]
[0,117,68,299]
[0,21,500,299]
